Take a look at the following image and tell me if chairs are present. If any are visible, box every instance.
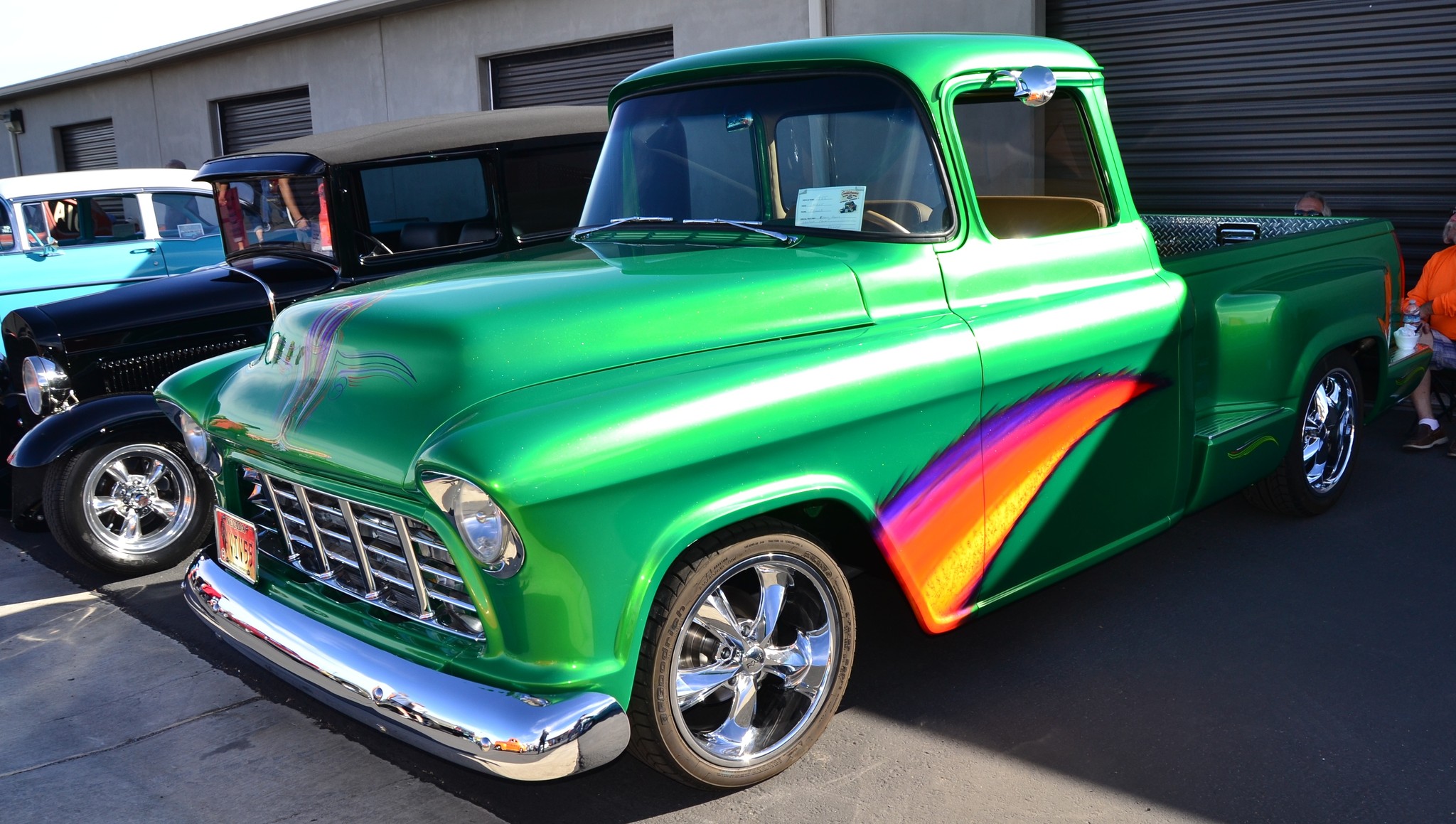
[392,215,523,249]
[795,193,1110,244]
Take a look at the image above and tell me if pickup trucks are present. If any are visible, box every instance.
[152,23,1434,807]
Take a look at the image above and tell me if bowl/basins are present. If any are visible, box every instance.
[1394,331,1420,350]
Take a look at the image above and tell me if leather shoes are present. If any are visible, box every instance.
[1403,422,1449,450]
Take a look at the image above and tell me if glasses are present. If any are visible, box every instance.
[1448,207,1456,218]
[1292,210,1323,216]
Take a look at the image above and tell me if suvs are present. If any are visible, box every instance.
[1,189,112,252]
[0,102,694,589]
[0,166,326,388]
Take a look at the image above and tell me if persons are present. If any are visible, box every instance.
[1400,212,1456,450]
[162,158,199,229]
[217,180,265,252]
[277,177,322,250]
[259,178,289,223]
[1292,193,1331,217]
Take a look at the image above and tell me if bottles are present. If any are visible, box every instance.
[1401,300,1422,343]
